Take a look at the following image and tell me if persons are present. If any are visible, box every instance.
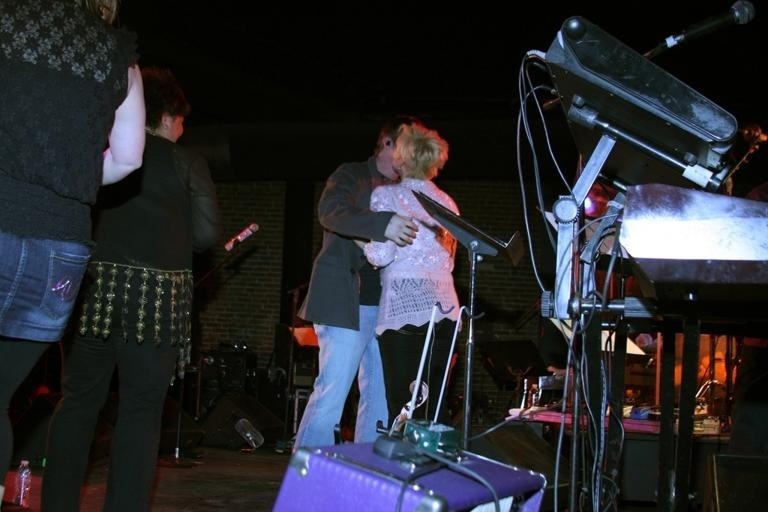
[292,115,421,451]
[39,80,228,512]
[0,0,148,505]
[354,123,460,431]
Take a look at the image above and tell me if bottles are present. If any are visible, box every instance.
[15,460,32,507]
[527,384,538,407]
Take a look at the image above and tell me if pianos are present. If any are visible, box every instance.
[548,18,738,189]
[617,181,768,288]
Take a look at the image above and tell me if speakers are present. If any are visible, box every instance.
[273,442,546,512]
[711,454,768,512]
[199,342,258,417]
[193,396,283,450]
[620,433,660,503]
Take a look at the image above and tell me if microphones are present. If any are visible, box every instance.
[224,223,259,251]
[674,0,756,43]
